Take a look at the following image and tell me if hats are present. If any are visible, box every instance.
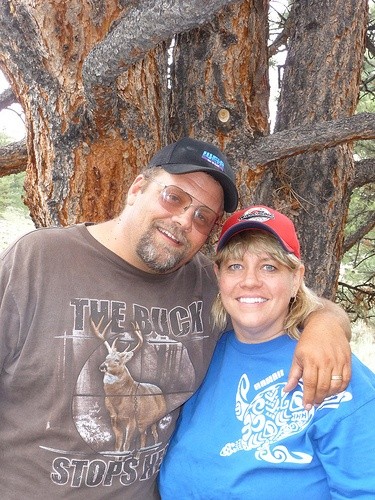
[216,205,300,259]
[146,138,239,213]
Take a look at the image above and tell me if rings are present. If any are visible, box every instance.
[331,376,342,379]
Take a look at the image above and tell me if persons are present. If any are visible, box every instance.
[0,137,353,500]
[159,209,375,500]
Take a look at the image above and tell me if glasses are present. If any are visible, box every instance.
[145,176,225,237]
